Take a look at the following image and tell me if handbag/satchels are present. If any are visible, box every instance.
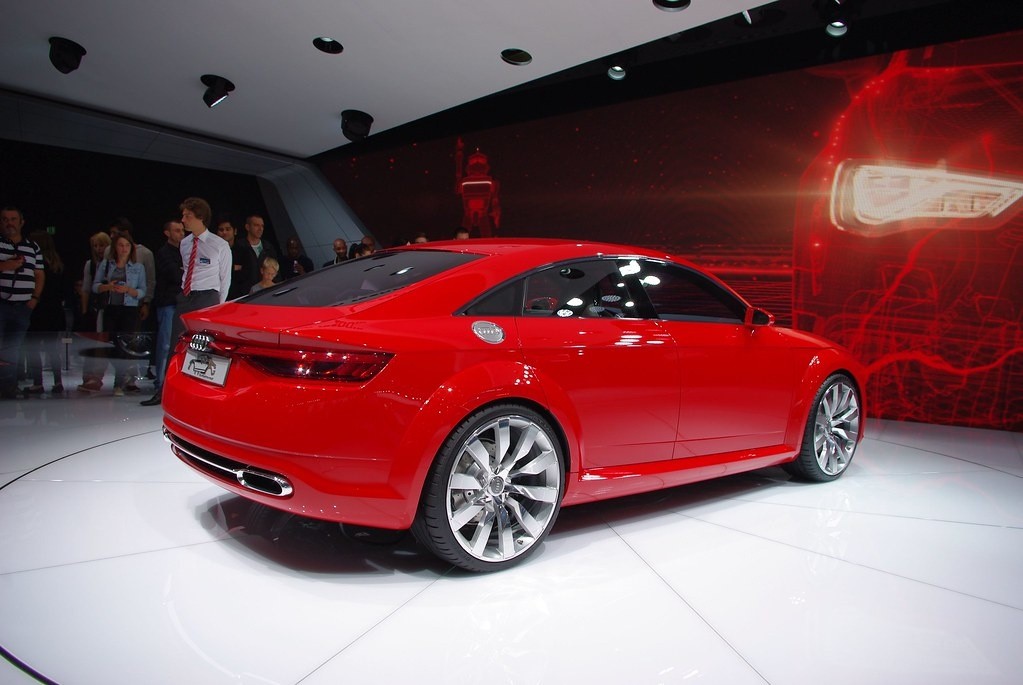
[89,259,109,308]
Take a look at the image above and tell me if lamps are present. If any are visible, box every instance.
[341,110,373,142]
[607,50,633,80]
[825,5,854,37]
[203,79,228,108]
[48,42,82,75]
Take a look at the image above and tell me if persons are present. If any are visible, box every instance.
[0,206,46,398]
[230,213,277,299]
[322,235,377,268]
[415,232,431,243]
[152,219,184,391]
[217,214,238,246]
[77,217,156,396]
[451,225,469,239]
[23,231,67,394]
[280,236,314,278]
[249,257,280,293]
[139,198,233,405]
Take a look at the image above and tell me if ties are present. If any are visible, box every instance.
[181,237,199,296]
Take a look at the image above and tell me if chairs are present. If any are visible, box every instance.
[549,275,614,318]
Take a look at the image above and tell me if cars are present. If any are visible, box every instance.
[160,236,867,575]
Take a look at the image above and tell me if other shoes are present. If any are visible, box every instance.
[78,375,103,392]
[23,386,44,394]
[51,384,64,393]
[2,384,23,398]
[113,386,124,396]
[140,390,161,406]
[124,385,143,393]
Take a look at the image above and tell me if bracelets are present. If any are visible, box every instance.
[31,294,40,303]
